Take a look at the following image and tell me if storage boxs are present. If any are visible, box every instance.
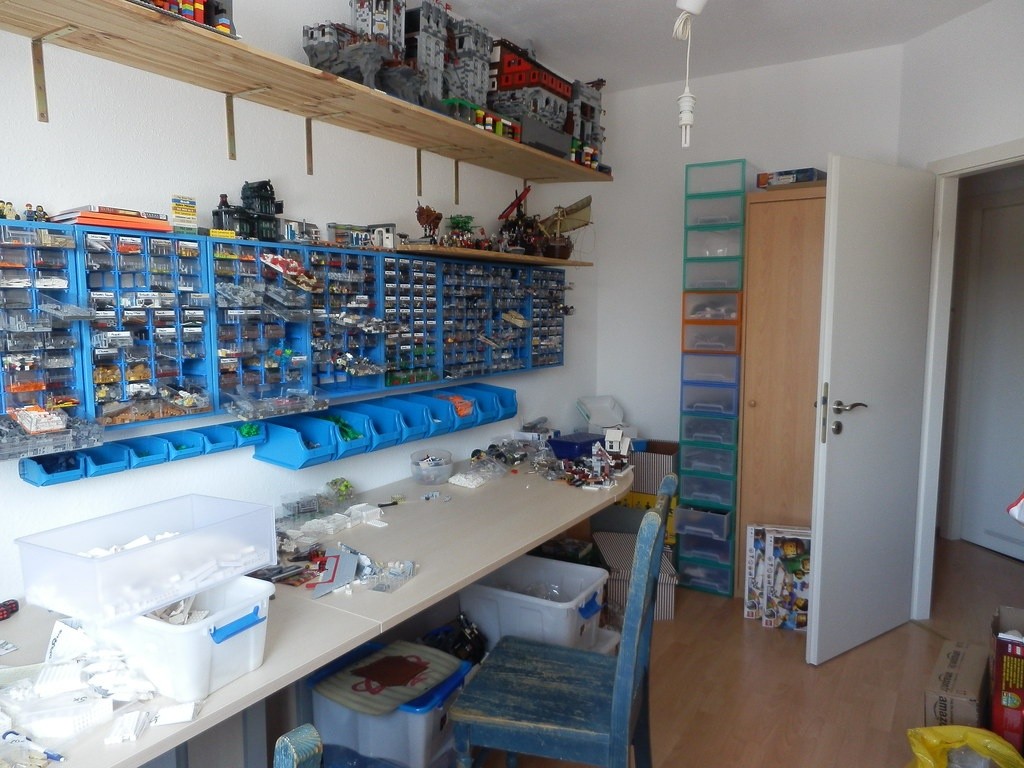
[920,640,994,733]
[744,523,813,621]
[18,383,518,488]
[760,529,813,632]
[756,167,828,191]
[308,642,475,768]
[576,158,767,621]
[82,573,277,708]
[457,552,610,656]
[14,495,279,626]
[989,604,1024,758]
[586,624,622,658]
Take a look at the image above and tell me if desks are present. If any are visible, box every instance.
[0,437,636,768]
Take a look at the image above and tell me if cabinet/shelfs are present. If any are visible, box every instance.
[0,219,564,461]
[730,179,828,600]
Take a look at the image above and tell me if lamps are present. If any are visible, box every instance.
[671,0,708,149]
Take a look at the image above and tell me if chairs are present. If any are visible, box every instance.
[444,472,680,768]
